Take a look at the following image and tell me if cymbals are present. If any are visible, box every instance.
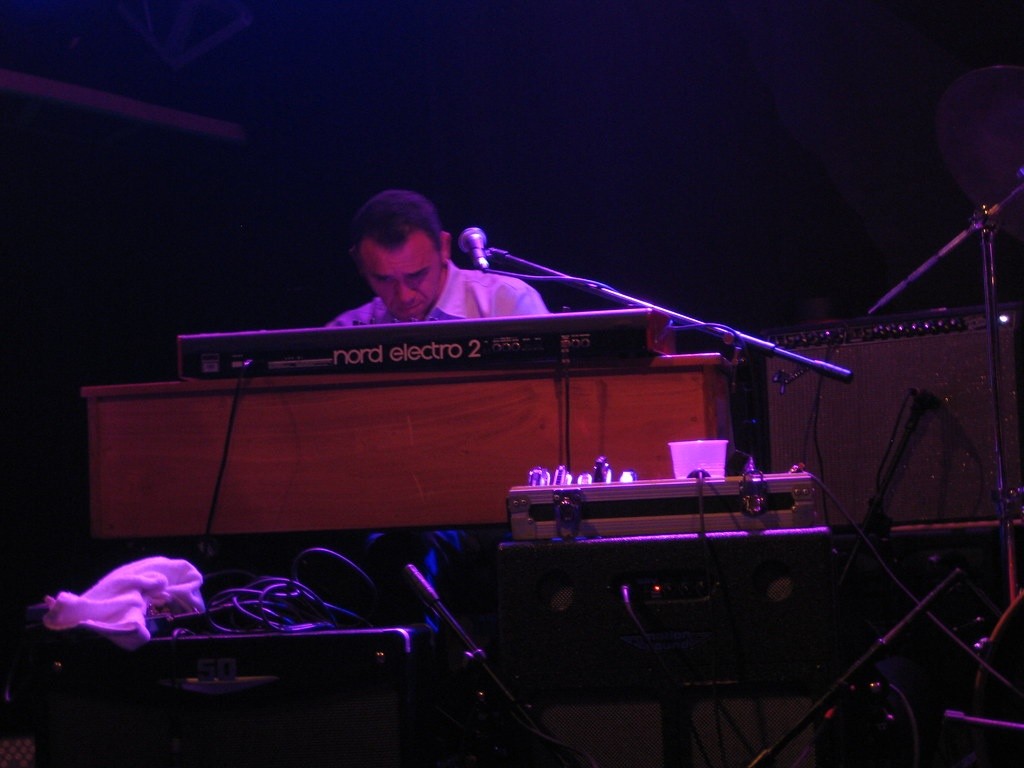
[934,65,1024,241]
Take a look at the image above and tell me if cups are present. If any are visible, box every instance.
[668,440,729,478]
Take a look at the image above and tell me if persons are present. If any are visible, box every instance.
[320,190,550,635]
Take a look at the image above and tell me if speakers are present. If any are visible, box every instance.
[519,695,845,768]
[761,300,1024,527]
[35,623,441,768]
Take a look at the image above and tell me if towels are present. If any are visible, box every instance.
[41,555,206,647]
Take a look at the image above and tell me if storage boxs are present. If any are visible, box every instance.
[508,470,817,542]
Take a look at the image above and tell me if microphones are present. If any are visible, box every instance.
[458,227,489,272]
[907,386,940,406]
